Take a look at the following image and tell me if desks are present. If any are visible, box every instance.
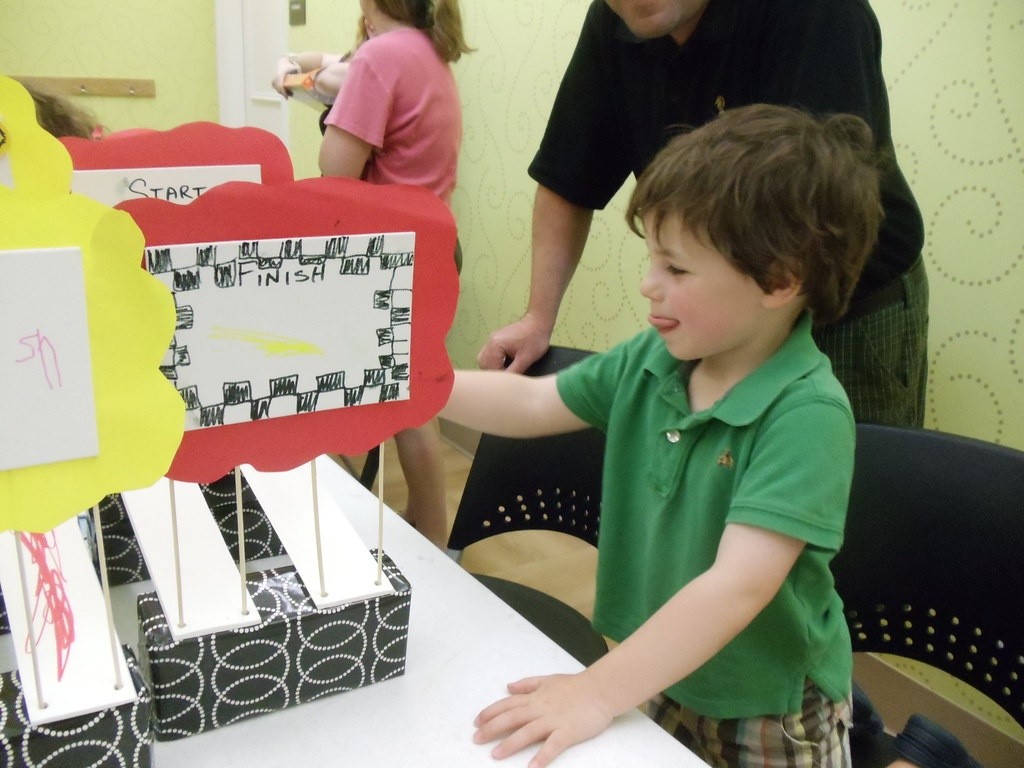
[1,454,714,768]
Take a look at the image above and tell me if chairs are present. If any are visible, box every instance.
[826,422,1023,768]
[445,344,609,672]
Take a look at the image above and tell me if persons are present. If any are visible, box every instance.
[271,0,477,549]
[436,107,983,768]
[476,0,929,428]
